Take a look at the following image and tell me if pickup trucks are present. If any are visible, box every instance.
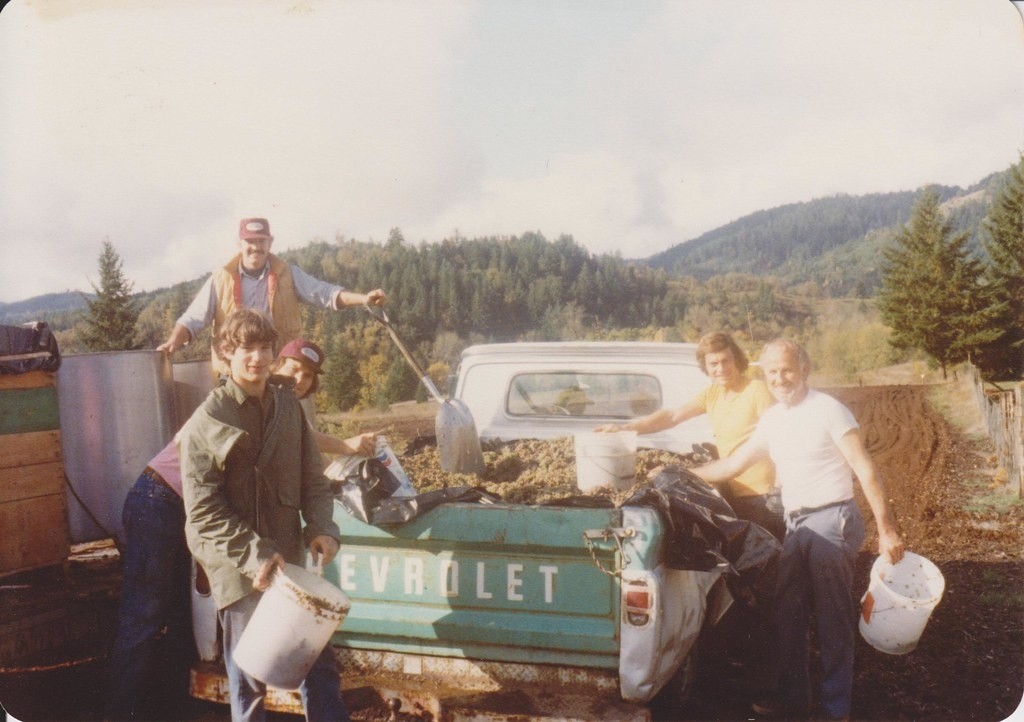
[187,340,736,722]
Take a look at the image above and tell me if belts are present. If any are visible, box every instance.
[142,465,167,488]
[789,497,848,518]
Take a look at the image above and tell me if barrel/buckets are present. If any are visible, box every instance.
[573,429,638,497]
[60,346,177,557]
[856,553,945,656]
[170,358,219,434]
[232,562,352,690]
[321,435,419,502]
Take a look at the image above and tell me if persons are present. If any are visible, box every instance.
[153,216,388,439]
[592,328,788,615]
[120,338,326,722]
[174,303,356,722]
[646,336,905,722]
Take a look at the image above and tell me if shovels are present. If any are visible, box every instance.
[363,302,490,481]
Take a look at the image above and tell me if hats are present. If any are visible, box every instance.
[277,338,325,375]
[238,218,270,239]
